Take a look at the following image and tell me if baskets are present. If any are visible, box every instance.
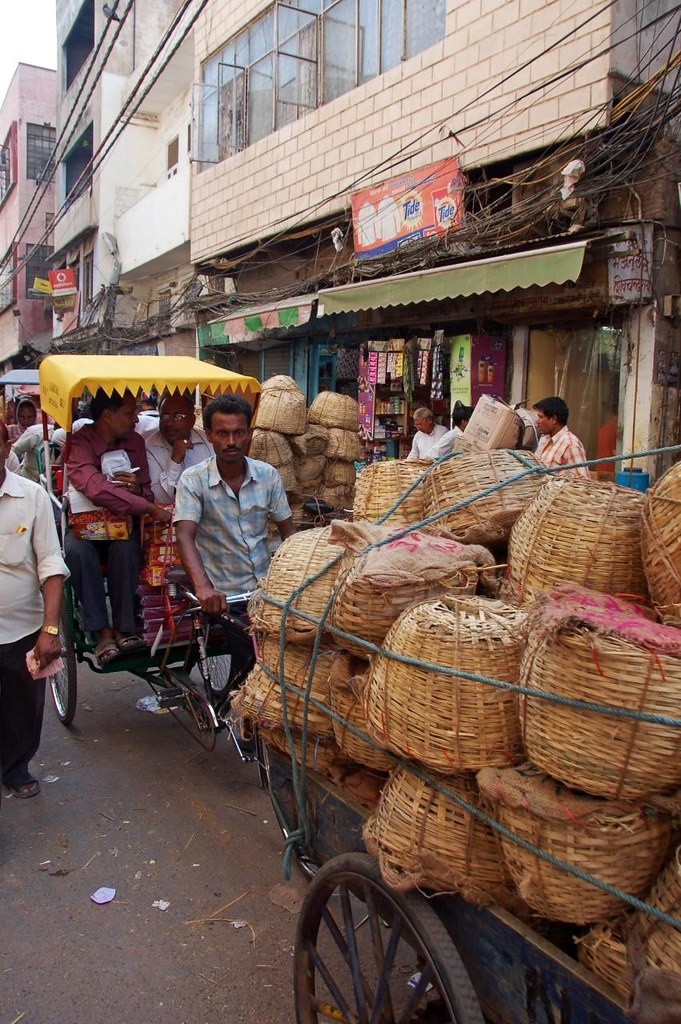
[238,375,681,997]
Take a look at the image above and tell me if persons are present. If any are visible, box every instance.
[0,419,71,798]
[61,386,171,665]
[406,406,474,460]
[532,396,591,481]
[146,392,216,504]
[172,395,297,753]
[5,389,160,484]
[594,402,619,472]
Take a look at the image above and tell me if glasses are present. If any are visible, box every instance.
[160,413,190,423]
[414,419,428,427]
[19,417,34,421]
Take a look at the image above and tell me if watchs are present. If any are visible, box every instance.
[41,625,59,636]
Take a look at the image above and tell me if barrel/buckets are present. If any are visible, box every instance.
[615,467,650,494]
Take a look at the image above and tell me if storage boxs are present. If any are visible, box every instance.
[139,506,184,588]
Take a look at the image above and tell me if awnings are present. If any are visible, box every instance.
[316,232,623,320]
[197,292,319,348]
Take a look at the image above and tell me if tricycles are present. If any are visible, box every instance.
[236,722,641,1024]
[30,354,326,883]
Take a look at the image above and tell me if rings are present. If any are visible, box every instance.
[183,440,188,444]
[128,483,131,487]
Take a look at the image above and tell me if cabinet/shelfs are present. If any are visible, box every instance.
[359,343,407,438]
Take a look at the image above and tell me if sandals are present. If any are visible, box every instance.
[6,772,41,798]
[95,632,145,664]
